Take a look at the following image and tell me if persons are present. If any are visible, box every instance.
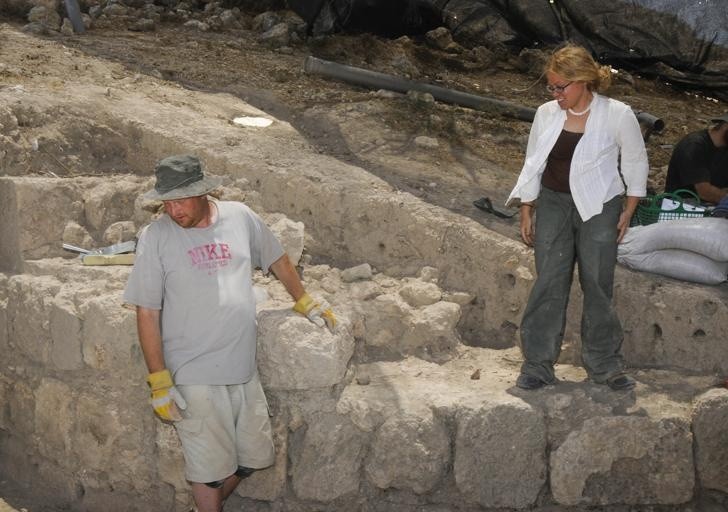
[664,110,728,206]
[504,43,651,391]
[119,151,339,512]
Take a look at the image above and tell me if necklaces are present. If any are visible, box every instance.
[568,105,590,116]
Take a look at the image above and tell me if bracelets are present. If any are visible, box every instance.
[520,203,534,208]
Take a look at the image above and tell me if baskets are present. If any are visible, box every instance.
[636,187,708,227]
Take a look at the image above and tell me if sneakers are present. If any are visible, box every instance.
[516,370,548,390]
[605,371,636,391]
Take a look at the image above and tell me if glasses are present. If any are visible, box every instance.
[545,80,575,94]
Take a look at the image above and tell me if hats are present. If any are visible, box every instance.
[141,151,225,201]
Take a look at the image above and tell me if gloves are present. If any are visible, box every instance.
[146,369,188,423]
[292,292,340,335]
